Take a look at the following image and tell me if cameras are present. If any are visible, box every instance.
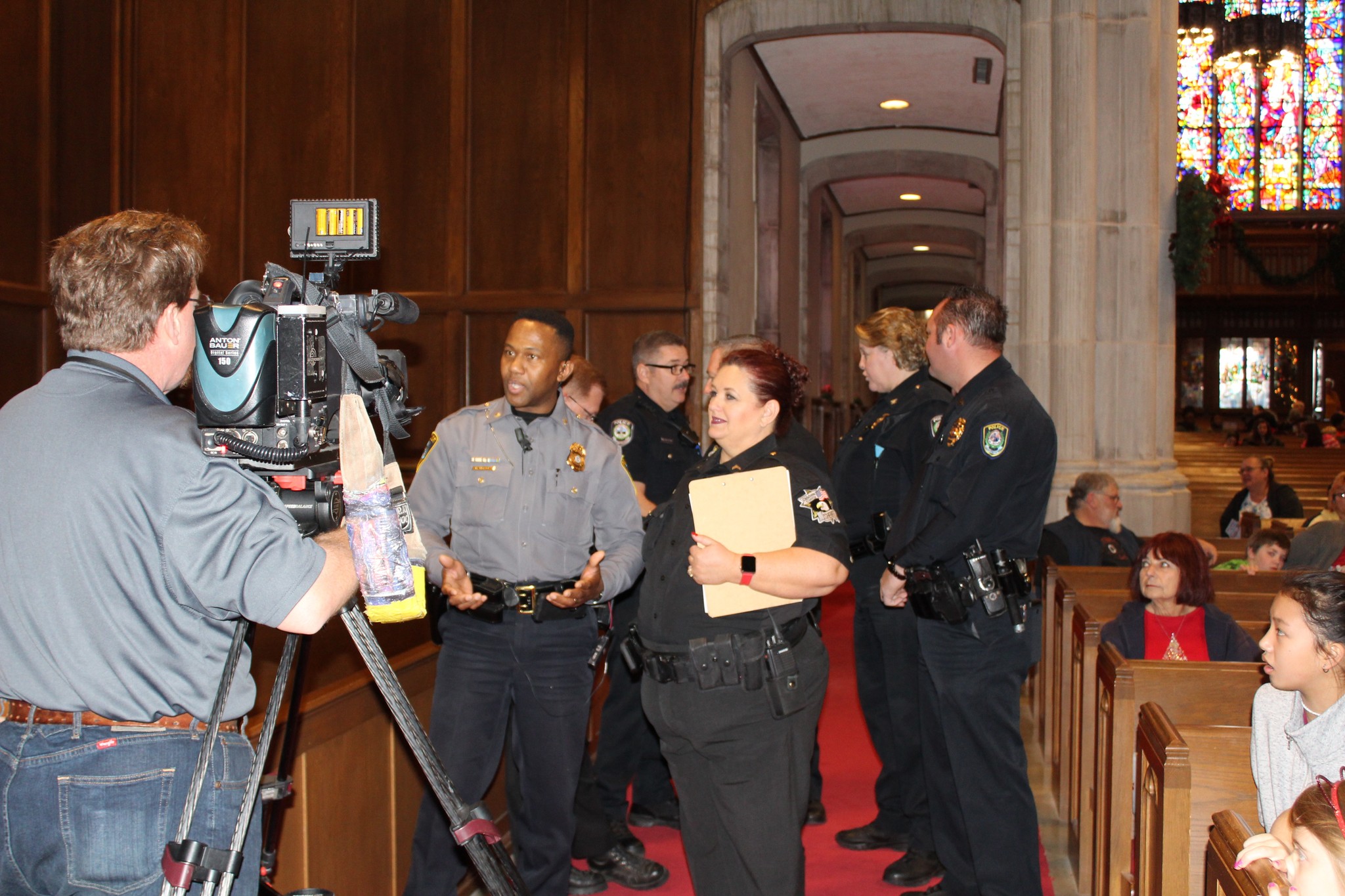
[849,512,892,560]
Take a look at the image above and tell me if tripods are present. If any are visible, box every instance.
[160,460,529,896]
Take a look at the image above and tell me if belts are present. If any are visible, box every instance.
[634,615,808,683]
[504,581,576,615]
[847,538,884,559]
[7,700,239,733]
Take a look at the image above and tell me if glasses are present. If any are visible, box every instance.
[567,395,595,422]
[1333,492,1345,499]
[645,364,696,375]
[1093,490,1119,505]
[1238,466,1262,475]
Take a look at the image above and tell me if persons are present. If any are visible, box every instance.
[836,309,955,887]
[879,284,1059,896]
[1250,571,1345,835]
[1101,531,1262,662]
[1220,455,1304,538]
[0,209,359,896]
[706,334,828,826]
[1235,766,1345,896]
[594,331,703,826]
[1038,471,1218,597]
[1180,403,1345,451]
[1210,528,1292,576]
[632,348,853,896]
[561,354,670,896]
[405,306,645,896]
[1283,469,1345,573]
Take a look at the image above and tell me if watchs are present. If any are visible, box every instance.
[740,552,756,587]
[886,557,907,581]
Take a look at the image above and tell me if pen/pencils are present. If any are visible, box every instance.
[696,442,700,449]
[556,468,560,486]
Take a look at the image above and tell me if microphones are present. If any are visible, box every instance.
[521,439,532,450]
[372,293,420,326]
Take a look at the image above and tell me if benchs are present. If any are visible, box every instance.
[1025,433,1345,896]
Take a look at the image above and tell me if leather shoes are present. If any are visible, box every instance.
[899,883,950,896]
[804,802,826,825]
[882,848,945,888]
[613,827,646,857]
[835,821,911,851]
[629,800,681,829]
[586,845,670,891]
[568,866,608,896]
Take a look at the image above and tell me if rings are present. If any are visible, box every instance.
[690,573,693,577]
